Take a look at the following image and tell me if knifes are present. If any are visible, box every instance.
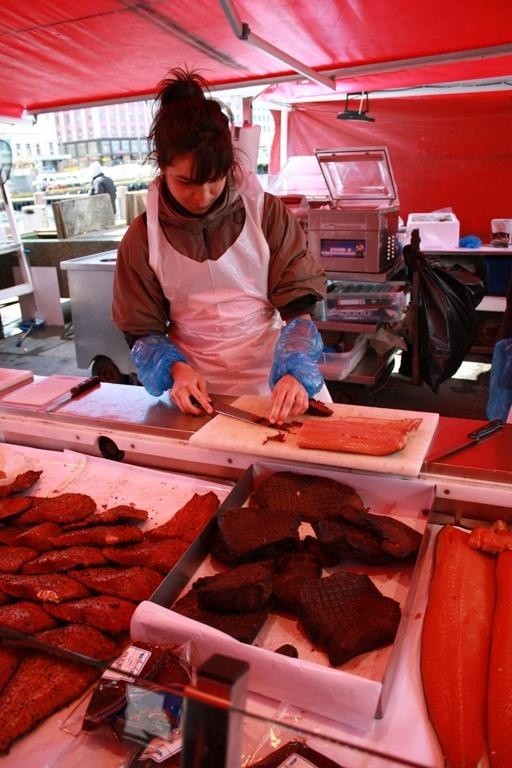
[193,397,288,433]
[425,419,505,462]
[42,376,101,411]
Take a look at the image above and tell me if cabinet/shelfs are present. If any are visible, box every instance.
[404,228,512,386]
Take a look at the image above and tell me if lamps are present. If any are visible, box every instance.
[335,92,377,123]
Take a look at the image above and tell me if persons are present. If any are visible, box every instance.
[110,61,329,426]
[89,161,116,215]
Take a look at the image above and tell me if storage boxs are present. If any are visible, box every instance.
[407,210,462,251]
[319,336,369,383]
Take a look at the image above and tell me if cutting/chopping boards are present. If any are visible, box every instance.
[190,393,440,478]
